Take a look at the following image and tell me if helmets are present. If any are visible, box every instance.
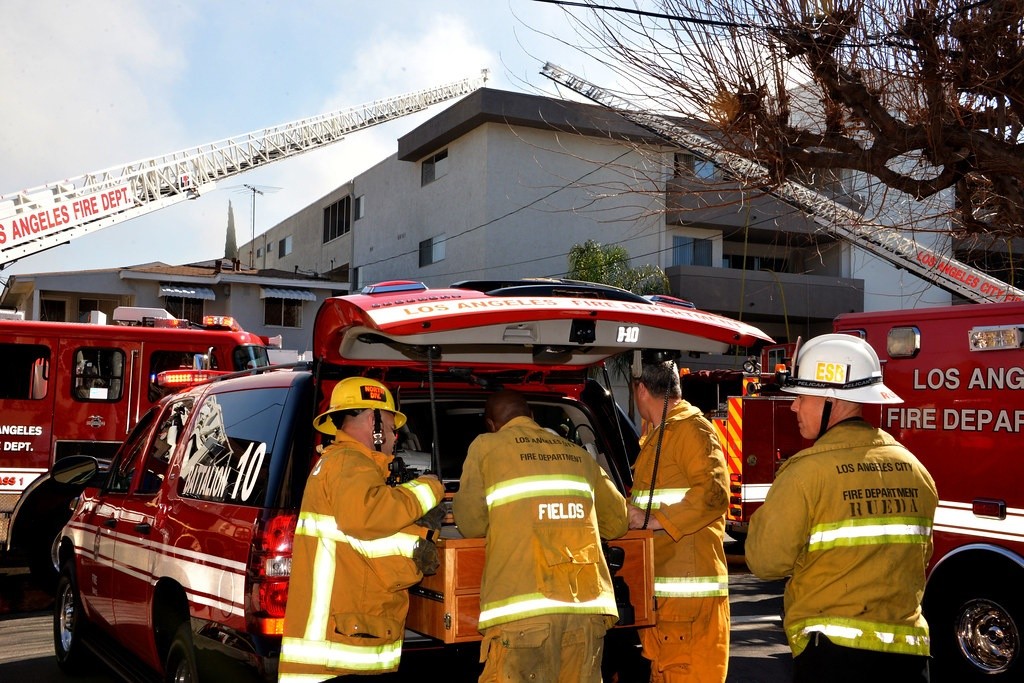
[313,376,407,435]
[779,333,905,404]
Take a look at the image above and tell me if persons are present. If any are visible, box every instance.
[277,376,447,683]
[451,388,628,683]
[627,358,731,683]
[745,332,939,683]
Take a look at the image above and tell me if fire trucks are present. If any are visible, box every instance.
[540,61,1023,683]
[48,276,778,683]
[684,335,815,536]
[0,66,477,591]
